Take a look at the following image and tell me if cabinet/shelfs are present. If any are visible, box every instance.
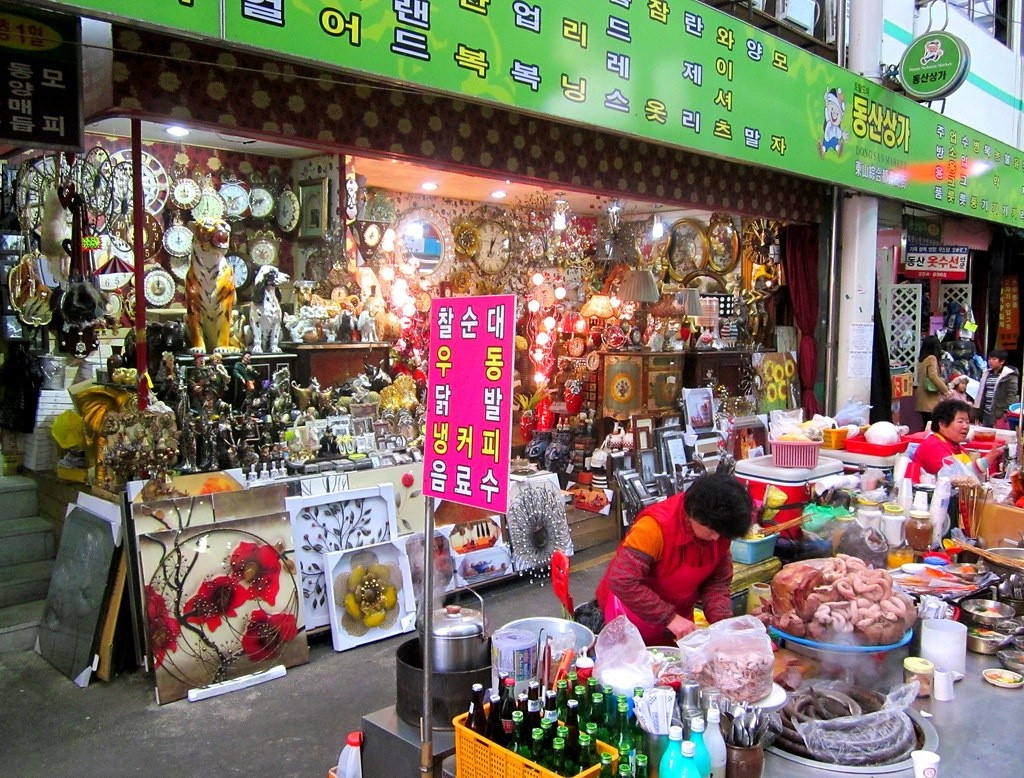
[599,347,775,441]
[178,343,390,424]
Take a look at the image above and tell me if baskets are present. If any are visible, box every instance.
[453,704,620,778]
[1009,402,1021,414]
[819,423,872,450]
[846,435,909,456]
[768,436,823,468]
[731,533,779,565]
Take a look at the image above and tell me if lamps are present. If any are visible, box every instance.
[579,270,735,351]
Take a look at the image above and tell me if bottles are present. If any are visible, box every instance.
[333,731,362,778]
[659,708,727,777]
[828,493,931,568]
[465,674,649,778]
[902,657,933,699]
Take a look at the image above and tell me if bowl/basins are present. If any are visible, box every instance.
[958,597,1024,675]
[493,617,597,657]
[942,561,989,581]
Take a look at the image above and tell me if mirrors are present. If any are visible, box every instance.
[393,207,455,288]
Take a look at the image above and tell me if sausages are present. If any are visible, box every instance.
[814,554,907,634]
[768,682,916,766]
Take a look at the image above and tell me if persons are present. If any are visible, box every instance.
[594,472,755,648]
[915,334,953,428]
[742,428,756,448]
[156,352,179,401]
[974,351,1019,428]
[209,353,232,399]
[902,397,1008,541]
[318,426,340,454]
[233,350,260,410]
[945,373,970,403]
[188,354,216,417]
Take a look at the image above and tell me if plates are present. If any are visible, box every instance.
[699,682,787,708]
[901,563,935,575]
[982,668,1024,688]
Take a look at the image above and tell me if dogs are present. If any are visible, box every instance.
[249,264,290,354]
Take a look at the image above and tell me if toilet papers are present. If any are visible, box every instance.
[933,666,954,702]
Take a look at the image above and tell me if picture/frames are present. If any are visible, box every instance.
[296,177,328,242]
[282,482,418,653]
[607,387,772,541]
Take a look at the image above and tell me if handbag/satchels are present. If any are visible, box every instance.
[923,377,945,392]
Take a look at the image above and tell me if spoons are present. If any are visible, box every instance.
[720,703,771,748]
[998,573,1023,601]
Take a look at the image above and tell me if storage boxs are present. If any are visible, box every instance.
[25,356,108,471]
[734,428,1024,547]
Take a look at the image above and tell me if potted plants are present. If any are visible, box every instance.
[514,386,549,443]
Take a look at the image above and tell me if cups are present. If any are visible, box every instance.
[921,618,967,680]
[898,476,953,538]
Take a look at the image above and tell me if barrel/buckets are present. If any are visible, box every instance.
[415,581,489,671]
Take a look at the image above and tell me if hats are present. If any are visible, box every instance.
[946,373,969,389]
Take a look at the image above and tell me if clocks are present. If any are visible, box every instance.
[471,220,511,275]
[742,218,782,265]
[568,338,601,371]
[415,291,433,314]
[15,149,391,306]
